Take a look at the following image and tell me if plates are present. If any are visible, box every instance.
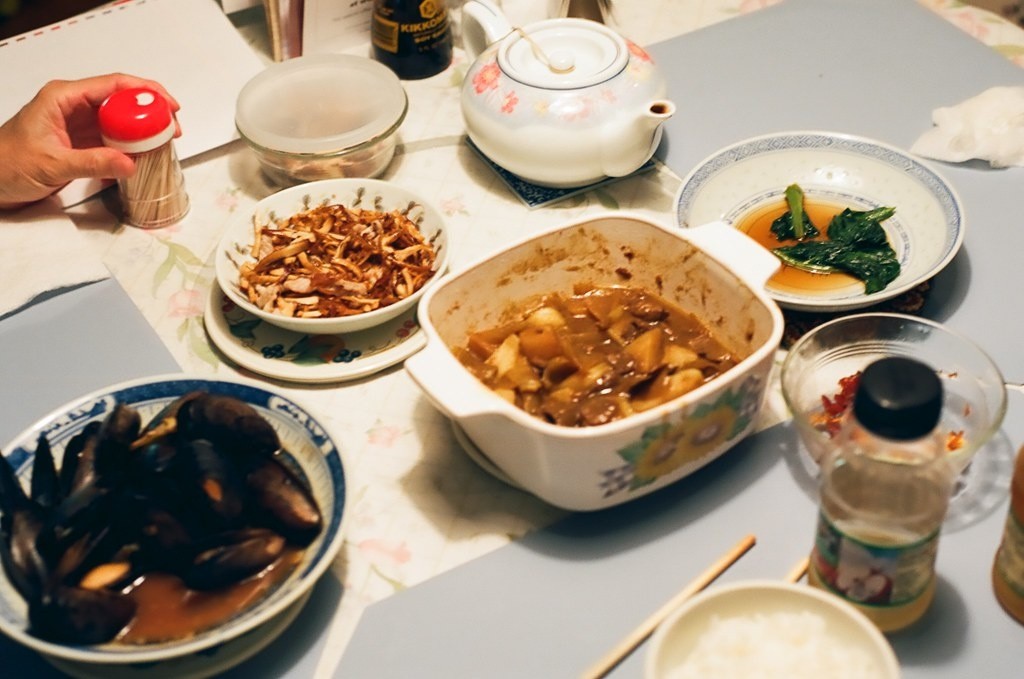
[447,354,791,486]
[641,584,902,679]
[463,137,657,208]
[32,585,311,679]
[213,178,453,334]
[0,372,352,679]
[675,130,965,311]
[203,262,466,384]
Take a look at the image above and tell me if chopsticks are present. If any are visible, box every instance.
[581,534,808,679]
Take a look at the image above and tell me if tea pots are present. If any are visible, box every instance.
[459,0,676,187]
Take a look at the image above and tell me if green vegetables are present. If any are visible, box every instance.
[771,183,903,296]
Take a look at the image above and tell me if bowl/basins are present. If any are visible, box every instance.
[404,214,784,514]
[780,309,1007,498]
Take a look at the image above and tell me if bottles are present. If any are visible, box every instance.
[808,354,950,636]
[991,444,1024,626]
[369,0,454,80]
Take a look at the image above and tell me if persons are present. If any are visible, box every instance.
[1,74,180,212]
